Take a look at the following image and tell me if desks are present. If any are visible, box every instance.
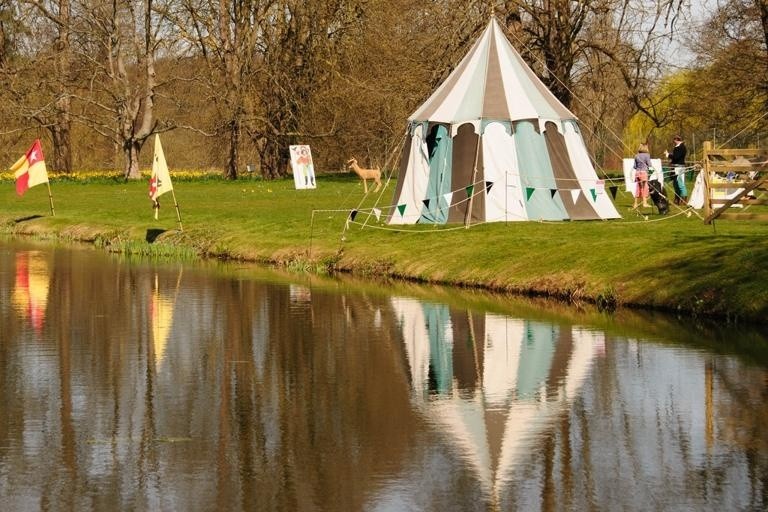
[711,175,753,208]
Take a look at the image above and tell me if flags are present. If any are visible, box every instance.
[10,250,53,340]
[149,291,175,371]
[0,137,49,197]
[148,132,172,203]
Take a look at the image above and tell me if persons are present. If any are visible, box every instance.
[291,146,315,186]
[728,155,752,183]
[633,133,688,209]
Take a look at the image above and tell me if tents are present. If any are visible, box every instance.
[383,3,624,225]
[389,294,606,512]
